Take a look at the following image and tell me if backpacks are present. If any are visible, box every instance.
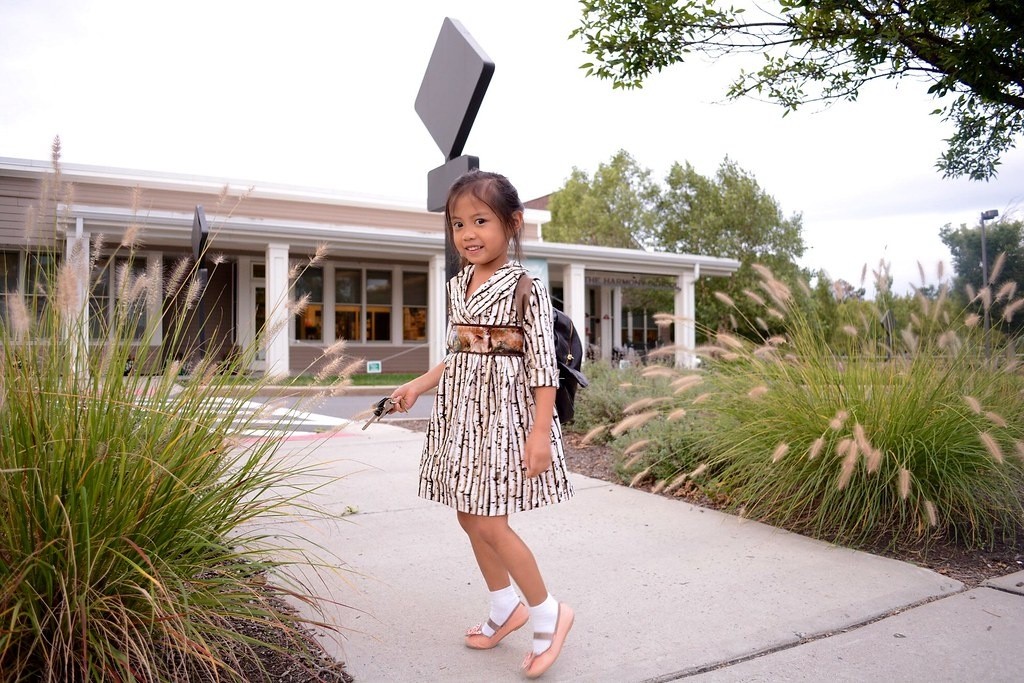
[516,273,589,424]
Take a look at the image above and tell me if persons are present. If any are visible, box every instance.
[388,172,575,679]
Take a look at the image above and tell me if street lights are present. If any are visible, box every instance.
[978,209,1000,368]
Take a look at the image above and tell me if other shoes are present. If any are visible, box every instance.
[464,600,530,650]
[523,601,574,679]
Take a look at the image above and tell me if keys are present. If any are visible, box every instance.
[399,399,408,413]
[362,397,394,430]
